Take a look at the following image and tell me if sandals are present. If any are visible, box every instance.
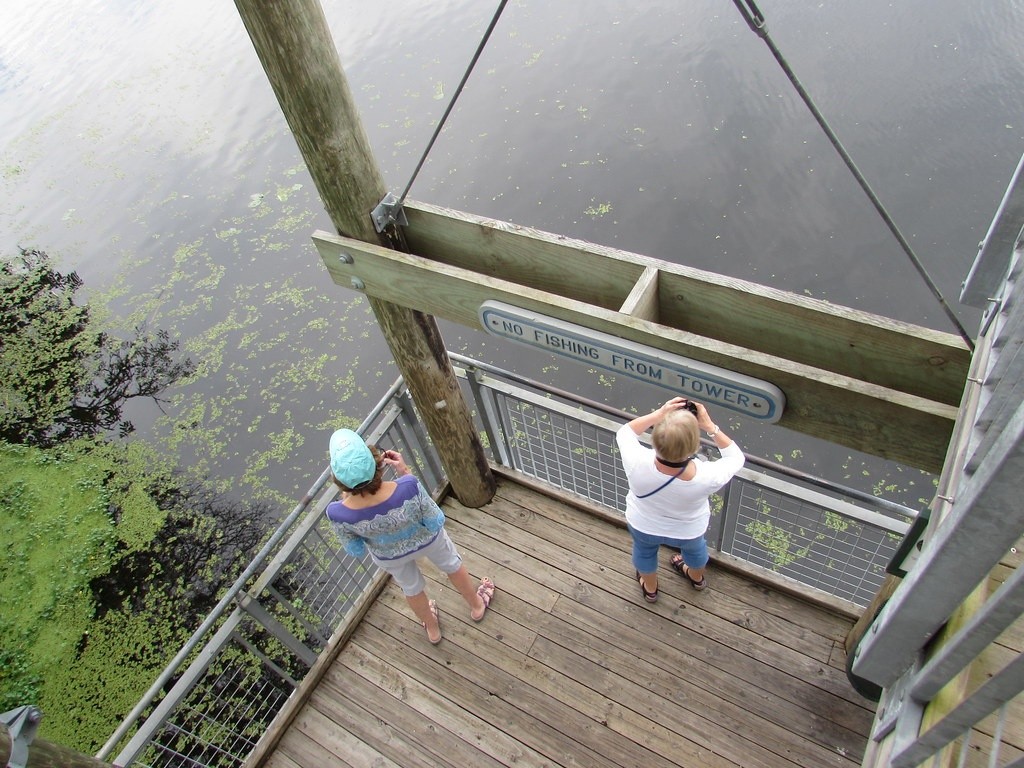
[670,553,706,590]
[636,570,659,602]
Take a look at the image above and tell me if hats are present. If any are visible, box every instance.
[329,428,376,489]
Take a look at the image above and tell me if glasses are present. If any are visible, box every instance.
[377,445,389,470]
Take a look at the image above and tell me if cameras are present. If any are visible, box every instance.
[679,399,697,415]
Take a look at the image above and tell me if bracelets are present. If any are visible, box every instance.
[706,424,720,437]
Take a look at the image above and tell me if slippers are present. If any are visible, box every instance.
[422,600,442,645]
[470,577,494,621]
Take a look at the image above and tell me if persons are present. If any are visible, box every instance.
[615,397,745,603]
[325,429,496,645]
